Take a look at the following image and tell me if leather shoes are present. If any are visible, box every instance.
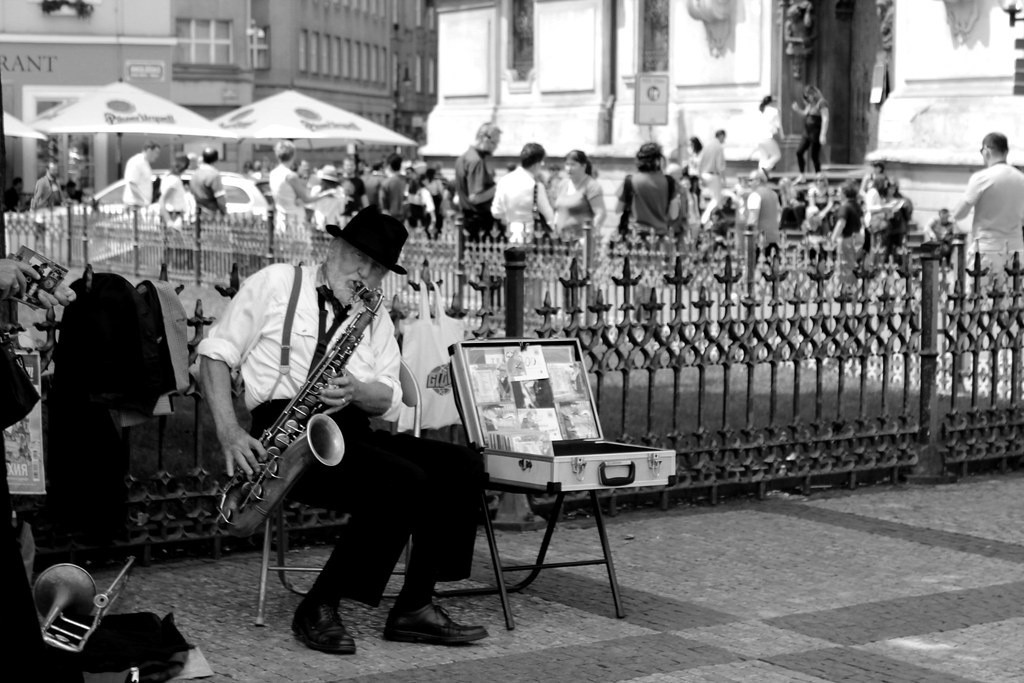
[292,599,356,654]
[383,600,488,643]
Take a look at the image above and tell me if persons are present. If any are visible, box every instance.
[191,203,490,655]
[4,176,24,212]
[0,255,86,683]
[31,260,48,282]
[29,156,63,210]
[753,95,786,184]
[453,122,607,332]
[949,131,1024,307]
[67,139,90,200]
[922,208,954,272]
[615,131,914,276]
[121,139,453,274]
[791,85,830,187]
[66,179,86,205]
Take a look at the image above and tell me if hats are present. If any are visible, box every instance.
[316,165,339,182]
[325,206,408,276]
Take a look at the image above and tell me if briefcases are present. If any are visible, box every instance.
[448,337,679,492]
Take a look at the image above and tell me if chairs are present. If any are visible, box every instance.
[449,360,624,629]
[257,360,422,626]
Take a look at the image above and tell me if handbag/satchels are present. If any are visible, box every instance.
[0,340,40,432]
[534,184,554,254]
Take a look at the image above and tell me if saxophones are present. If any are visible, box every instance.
[213,282,386,536]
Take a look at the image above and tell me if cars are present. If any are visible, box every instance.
[49,171,276,220]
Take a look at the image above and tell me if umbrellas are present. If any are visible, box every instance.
[214,87,420,151]
[4,110,49,141]
[25,76,239,181]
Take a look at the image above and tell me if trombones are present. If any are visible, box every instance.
[32,557,136,653]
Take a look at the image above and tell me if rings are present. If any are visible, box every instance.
[341,398,346,403]
